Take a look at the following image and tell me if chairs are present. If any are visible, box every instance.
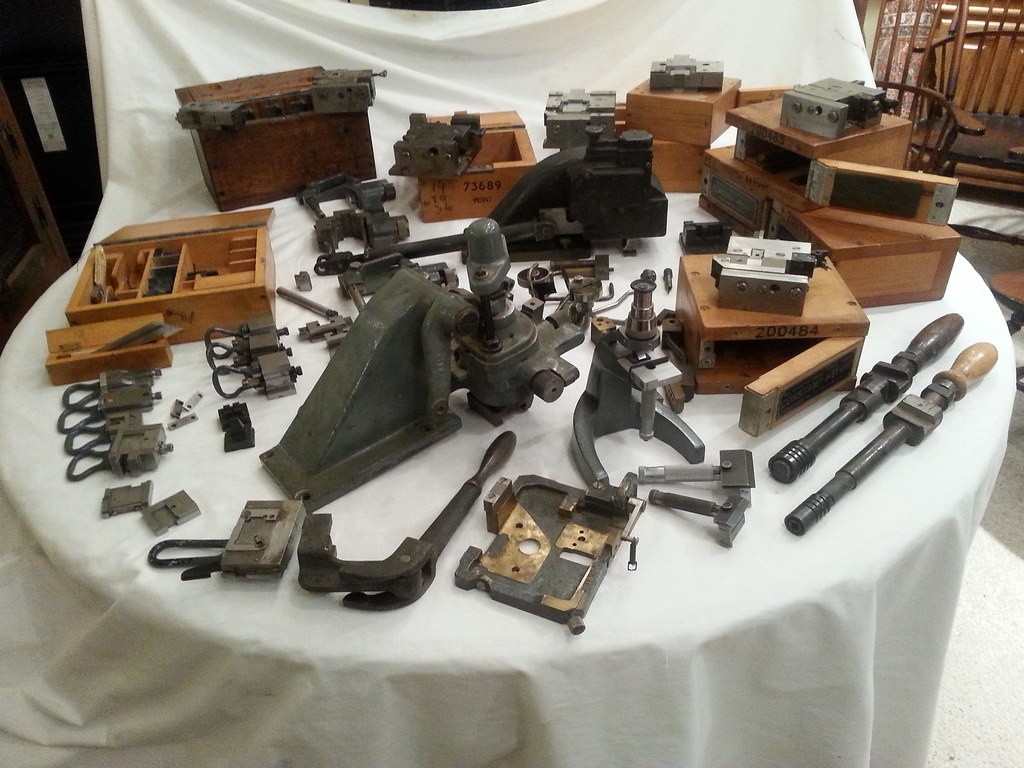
[874,80,958,176]
[915,26,1024,247]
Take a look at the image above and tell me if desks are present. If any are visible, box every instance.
[0,204,1017,767]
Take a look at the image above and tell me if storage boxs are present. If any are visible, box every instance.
[44,66,959,439]
